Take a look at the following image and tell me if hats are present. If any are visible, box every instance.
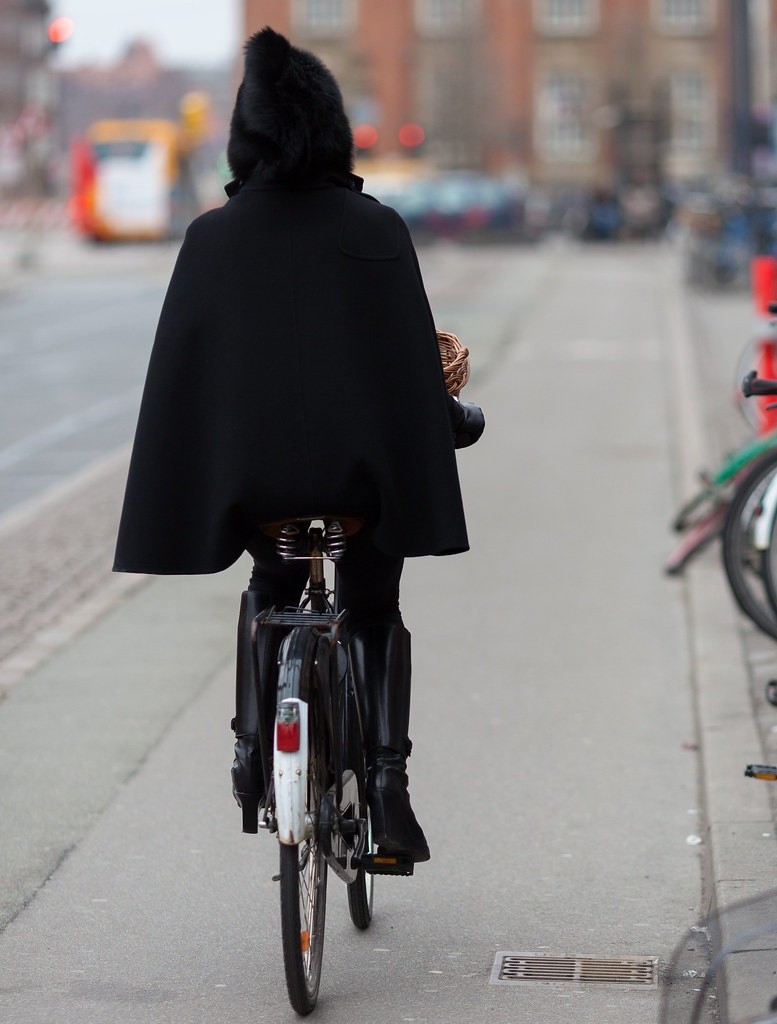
[228,26,354,187]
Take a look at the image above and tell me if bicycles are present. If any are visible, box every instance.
[661,302,777,784]
[236,327,470,1018]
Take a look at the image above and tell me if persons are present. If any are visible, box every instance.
[112,26,485,864]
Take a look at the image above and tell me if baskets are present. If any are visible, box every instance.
[437,333,469,398]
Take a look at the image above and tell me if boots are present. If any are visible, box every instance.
[348,620,435,863]
[232,587,281,834]
